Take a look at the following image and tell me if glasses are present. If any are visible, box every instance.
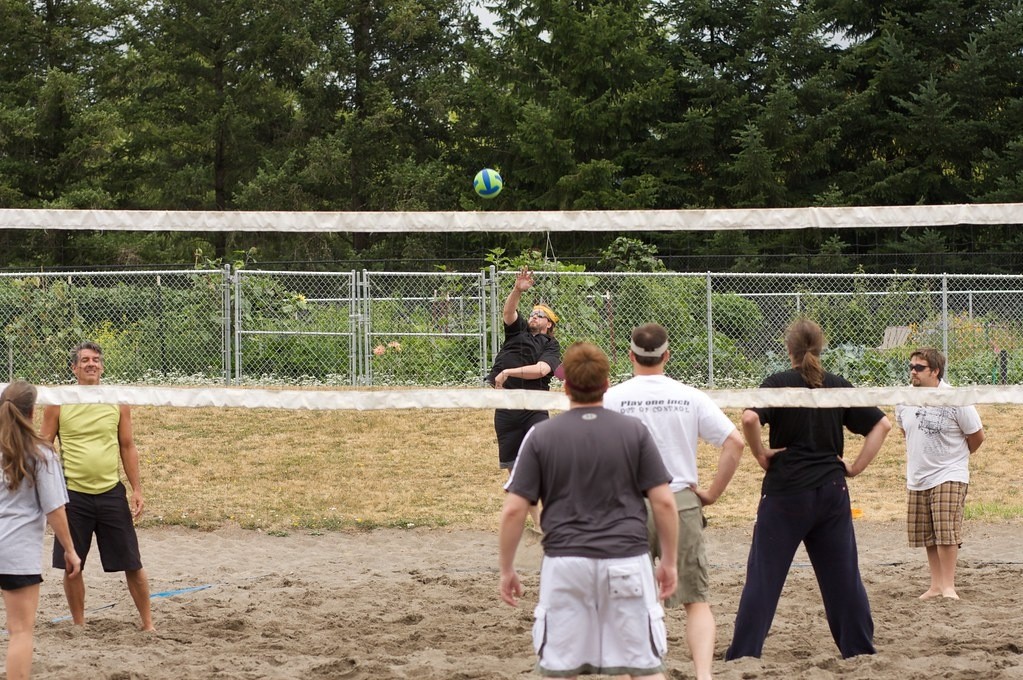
[909,363,931,372]
[530,312,550,321]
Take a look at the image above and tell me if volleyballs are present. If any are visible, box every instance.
[473,168,503,200]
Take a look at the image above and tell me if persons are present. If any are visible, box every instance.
[40,341,158,633]
[498,343,680,680]
[483,264,561,546]
[894,348,986,600]
[0,381,81,680]
[603,323,745,680]
[724,316,892,661]
